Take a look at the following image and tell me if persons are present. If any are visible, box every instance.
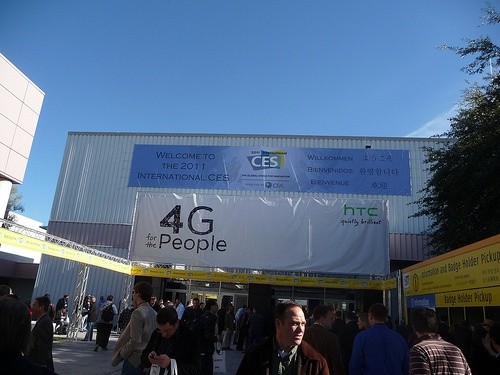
[410,307,471,375]
[0,280,500,375]
[235,301,330,375]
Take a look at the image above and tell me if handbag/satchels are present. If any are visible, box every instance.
[212,337,227,375]
[102,302,115,322]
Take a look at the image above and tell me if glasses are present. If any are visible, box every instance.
[157,327,174,335]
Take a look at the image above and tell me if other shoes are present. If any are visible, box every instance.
[101,347,109,351]
[94,345,99,352]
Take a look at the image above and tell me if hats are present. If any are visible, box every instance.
[480,320,495,327]
